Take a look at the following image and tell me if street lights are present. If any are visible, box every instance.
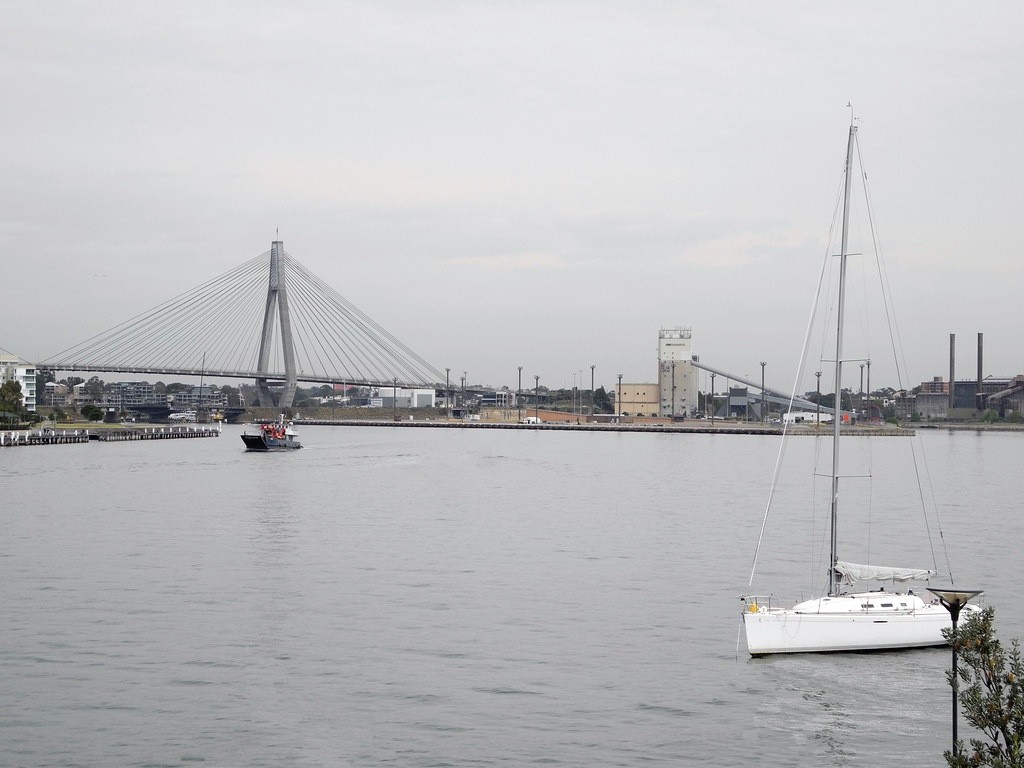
[926,588,984,757]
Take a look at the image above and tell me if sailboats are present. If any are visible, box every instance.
[736,97,991,659]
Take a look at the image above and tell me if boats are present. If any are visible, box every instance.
[240,413,304,453]
